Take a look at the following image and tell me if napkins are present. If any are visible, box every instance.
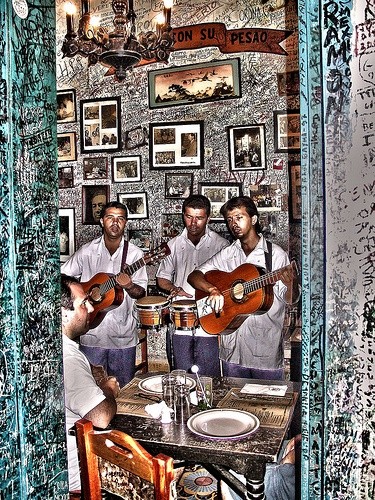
[145,401,173,424]
[240,382,288,396]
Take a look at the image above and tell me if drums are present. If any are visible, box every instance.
[136,296,170,329]
[172,300,199,330]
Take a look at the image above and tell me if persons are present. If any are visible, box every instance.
[219,433,310,499]
[55,87,281,249]
[186,196,297,384]
[57,200,148,396]
[61,272,155,500]
[153,193,229,378]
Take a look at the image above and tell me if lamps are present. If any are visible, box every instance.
[61,0,175,81]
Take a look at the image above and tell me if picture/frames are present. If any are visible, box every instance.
[56,89,77,124]
[59,208,76,262]
[149,57,242,109]
[117,192,148,220]
[58,166,73,189]
[272,109,301,153]
[149,120,205,170]
[56,132,76,161]
[111,156,142,183]
[228,123,267,172]
[161,160,302,248]
[82,185,110,226]
[82,157,108,180]
[277,70,300,96]
[80,96,122,153]
[126,229,154,252]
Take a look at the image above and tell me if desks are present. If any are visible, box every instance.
[69,372,302,500]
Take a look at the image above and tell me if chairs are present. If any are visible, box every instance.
[76,419,175,500]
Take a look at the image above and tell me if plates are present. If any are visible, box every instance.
[187,407,261,441]
[138,375,197,395]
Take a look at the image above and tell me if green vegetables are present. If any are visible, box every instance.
[198,400,207,409]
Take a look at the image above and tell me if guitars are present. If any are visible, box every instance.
[196,258,300,335]
[79,242,171,329]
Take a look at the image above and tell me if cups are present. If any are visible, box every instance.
[171,369,186,385]
[195,377,213,411]
[161,376,190,426]
